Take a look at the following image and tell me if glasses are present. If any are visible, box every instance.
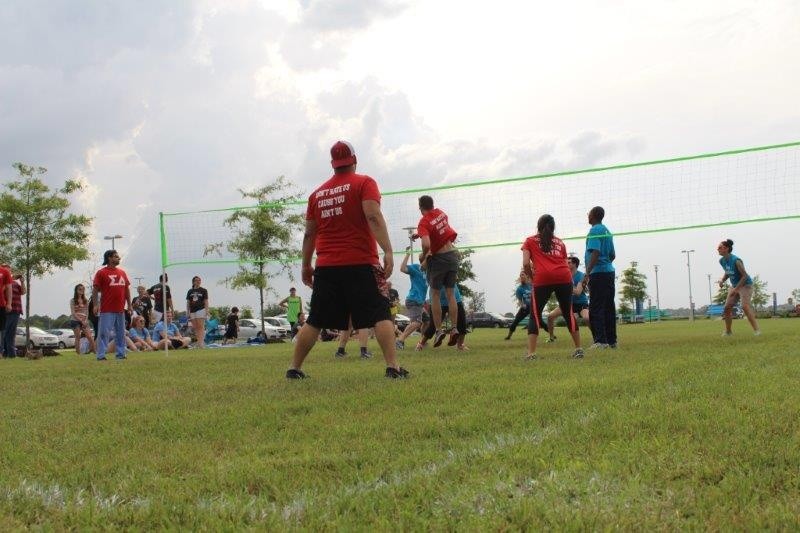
[140,320,145,322]
[113,254,119,258]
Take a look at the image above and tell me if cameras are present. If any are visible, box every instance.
[15,275,22,279]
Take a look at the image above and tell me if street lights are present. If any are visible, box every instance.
[402,227,417,264]
[104,234,122,250]
[681,250,695,306]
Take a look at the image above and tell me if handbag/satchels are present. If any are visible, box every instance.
[25,347,43,360]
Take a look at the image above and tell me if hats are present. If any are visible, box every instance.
[102,250,116,265]
[330,140,356,168]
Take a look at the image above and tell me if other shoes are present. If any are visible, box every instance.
[97,357,107,360]
[285,368,310,380]
[385,366,409,379]
[396,338,404,350]
[361,352,373,360]
[525,353,536,360]
[415,341,422,351]
[432,331,446,347]
[448,328,459,346]
[546,338,554,343]
[571,349,583,359]
[588,342,609,350]
[334,351,348,358]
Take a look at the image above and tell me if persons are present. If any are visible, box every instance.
[69,249,191,360]
[414,273,468,351]
[285,140,411,380]
[409,196,459,346]
[291,312,306,337]
[0,263,27,359]
[185,276,209,349]
[717,239,762,338]
[504,204,620,362]
[395,245,431,350]
[334,313,375,359]
[279,287,303,330]
[221,306,241,346]
[321,327,333,342]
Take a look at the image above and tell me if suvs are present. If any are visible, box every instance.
[466,311,508,329]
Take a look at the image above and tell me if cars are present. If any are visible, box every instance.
[14,327,76,353]
[220,314,293,341]
[706,305,743,320]
[395,315,410,334]
